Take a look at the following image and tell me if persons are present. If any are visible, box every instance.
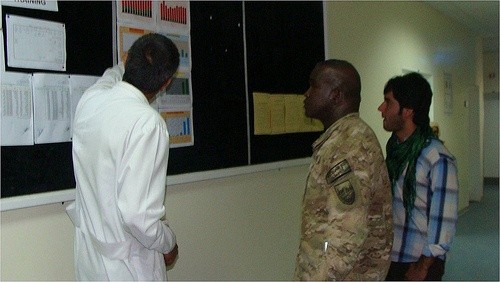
[64,32,183,282]
[376,70,461,282]
[293,58,395,282]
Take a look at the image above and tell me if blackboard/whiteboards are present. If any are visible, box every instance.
[0,0,332,214]
